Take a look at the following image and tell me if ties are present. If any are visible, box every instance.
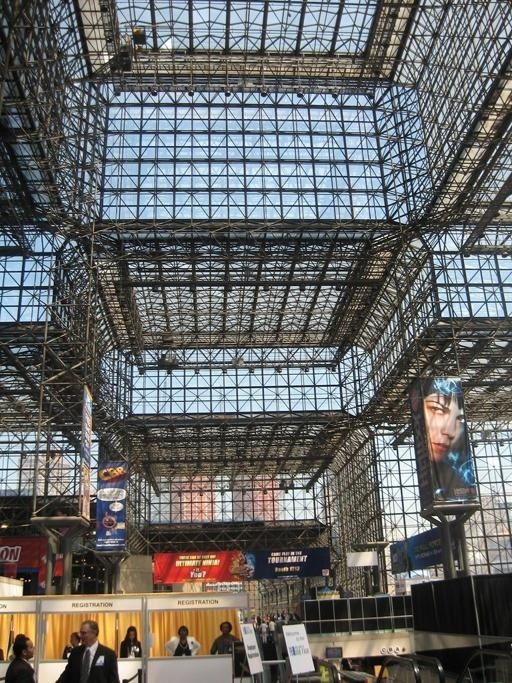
[81,649,90,682]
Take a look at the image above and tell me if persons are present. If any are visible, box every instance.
[162,624,201,656]
[206,620,241,655]
[116,624,140,658]
[411,375,467,498]
[243,609,300,645]
[2,636,38,683]
[60,630,81,659]
[52,618,119,682]
[5,634,25,662]
